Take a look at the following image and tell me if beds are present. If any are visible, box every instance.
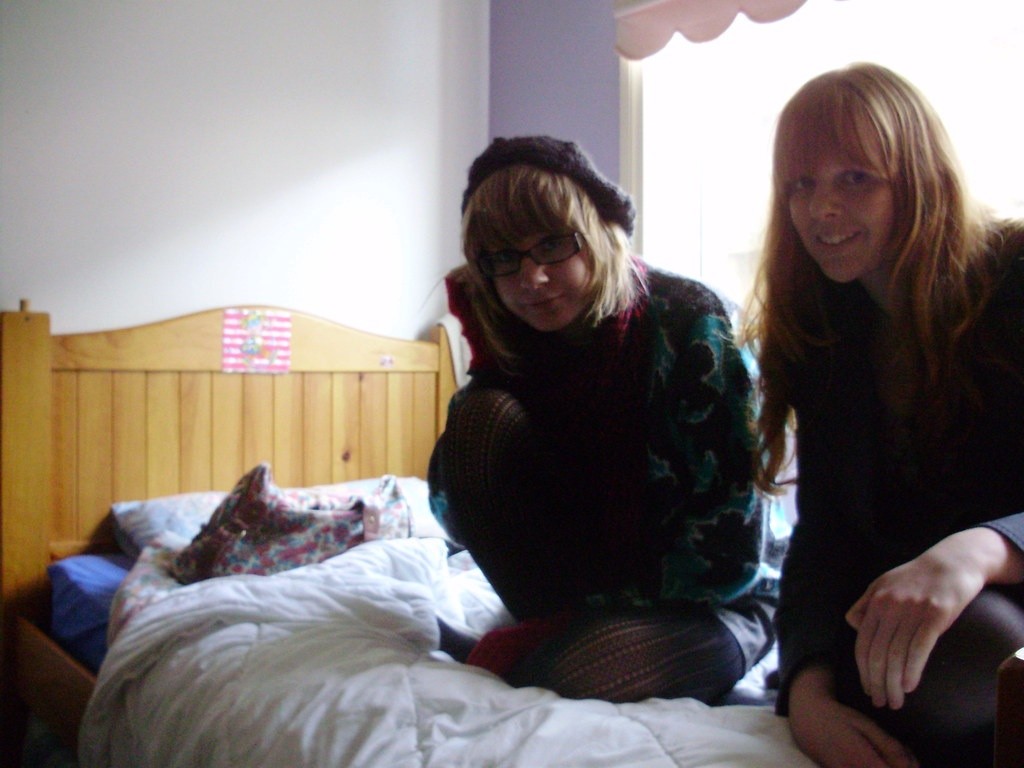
[16,479,825,768]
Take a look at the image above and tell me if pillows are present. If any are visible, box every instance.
[111,473,449,559]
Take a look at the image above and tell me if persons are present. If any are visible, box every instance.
[727,66,1024,768]
[427,133,778,709]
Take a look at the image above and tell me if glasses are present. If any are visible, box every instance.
[477,230,585,279]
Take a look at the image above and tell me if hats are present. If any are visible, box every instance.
[459,133,635,239]
